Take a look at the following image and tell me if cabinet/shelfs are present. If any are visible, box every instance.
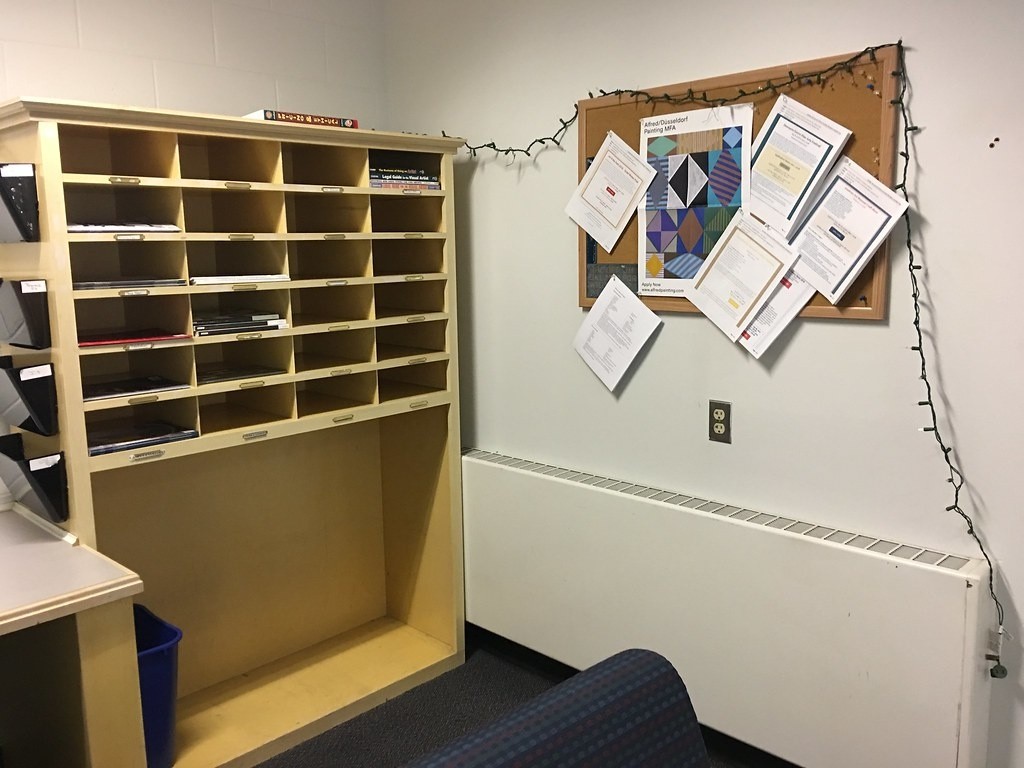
[0,100,468,768]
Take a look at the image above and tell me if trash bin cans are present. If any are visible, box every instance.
[132,602,182,768]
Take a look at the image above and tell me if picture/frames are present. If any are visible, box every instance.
[577,45,899,321]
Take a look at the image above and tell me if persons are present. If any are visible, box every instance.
[342,120,353,127]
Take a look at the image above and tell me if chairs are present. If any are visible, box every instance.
[404,649,709,768]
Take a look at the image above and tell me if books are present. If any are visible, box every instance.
[67,219,181,232]
[73,278,188,290]
[82,376,191,402]
[197,368,287,384]
[87,420,199,456]
[242,109,358,129]
[78,328,192,346]
[190,274,291,285]
[192,310,289,336]
[370,167,441,190]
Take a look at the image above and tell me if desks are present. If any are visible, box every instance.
[0,500,149,768]
